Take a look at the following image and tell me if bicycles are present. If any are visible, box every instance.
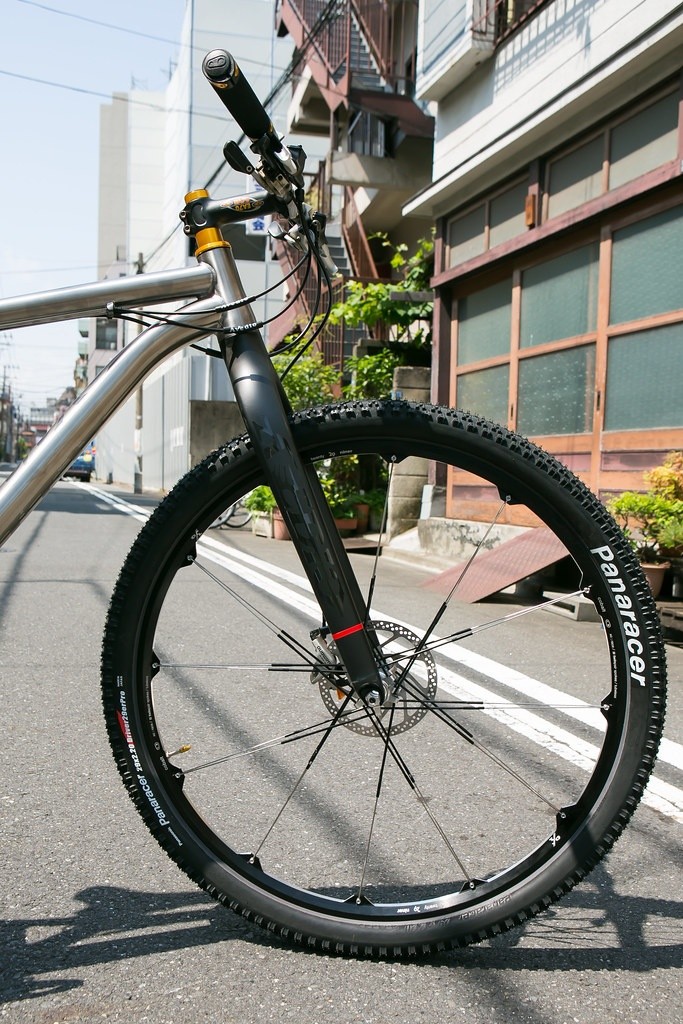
[1,46,669,964]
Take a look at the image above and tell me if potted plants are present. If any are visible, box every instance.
[249,334,403,540]
[605,450,683,602]
